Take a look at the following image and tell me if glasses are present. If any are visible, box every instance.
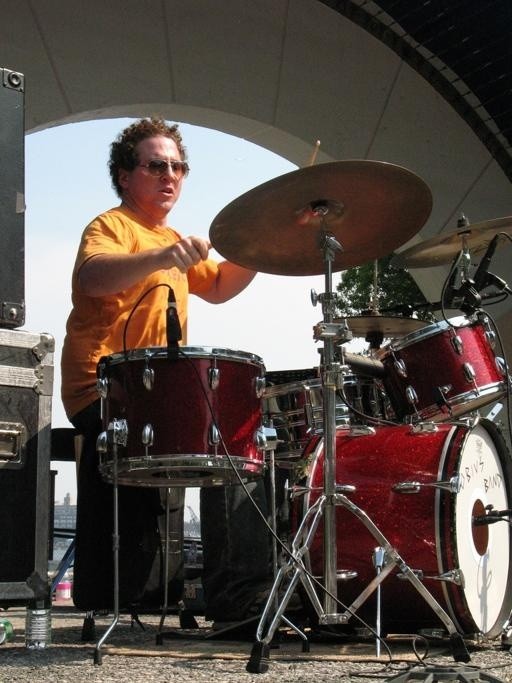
[137,158,191,179]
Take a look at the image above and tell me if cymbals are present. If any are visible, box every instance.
[315,315,432,337]
[208,159,433,276]
[390,215,512,267]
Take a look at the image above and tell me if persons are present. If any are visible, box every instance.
[62,117,258,611]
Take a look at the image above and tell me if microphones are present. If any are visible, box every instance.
[471,234,499,296]
[165,289,182,386]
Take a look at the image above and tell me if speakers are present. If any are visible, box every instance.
[0,68,27,329]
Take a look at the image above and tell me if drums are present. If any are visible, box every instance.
[262,375,376,465]
[374,310,511,424]
[289,416,511,647]
[98,344,266,489]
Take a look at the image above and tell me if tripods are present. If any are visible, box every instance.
[244,255,470,673]
